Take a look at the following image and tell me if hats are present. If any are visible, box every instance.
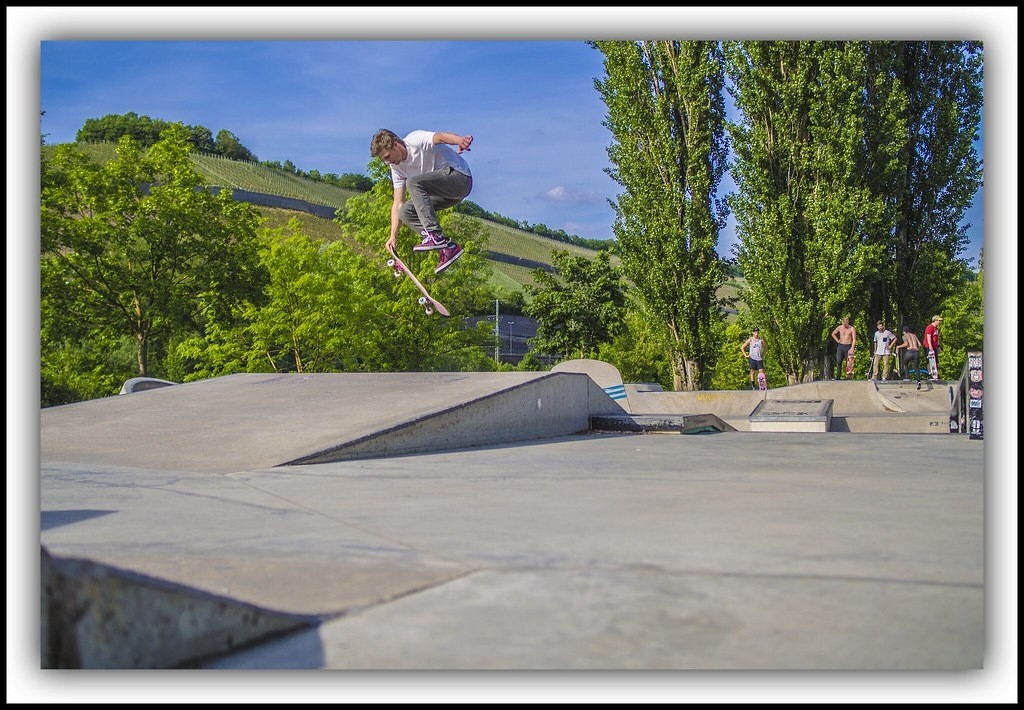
[753,327,760,332]
[932,315,943,322]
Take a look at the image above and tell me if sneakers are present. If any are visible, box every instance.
[434,241,464,273]
[413,231,448,251]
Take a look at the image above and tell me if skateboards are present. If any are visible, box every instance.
[387,244,451,317]
[846,353,856,379]
[892,346,901,377]
[757,373,768,391]
[927,349,938,379]
[866,355,875,379]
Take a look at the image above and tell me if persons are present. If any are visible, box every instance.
[897,326,922,390]
[923,315,943,381]
[370,129,474,273]
[868,321,897,383]
[832,318,856,380]
[741,327,767,390]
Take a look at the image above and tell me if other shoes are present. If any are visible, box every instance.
[881,378,888,384]
[753,387,758,390]
[832,377,840,381]
[917,382,921,391]
[868,377,878,382]
[928,375,940,381]
[903,377,911,382]
